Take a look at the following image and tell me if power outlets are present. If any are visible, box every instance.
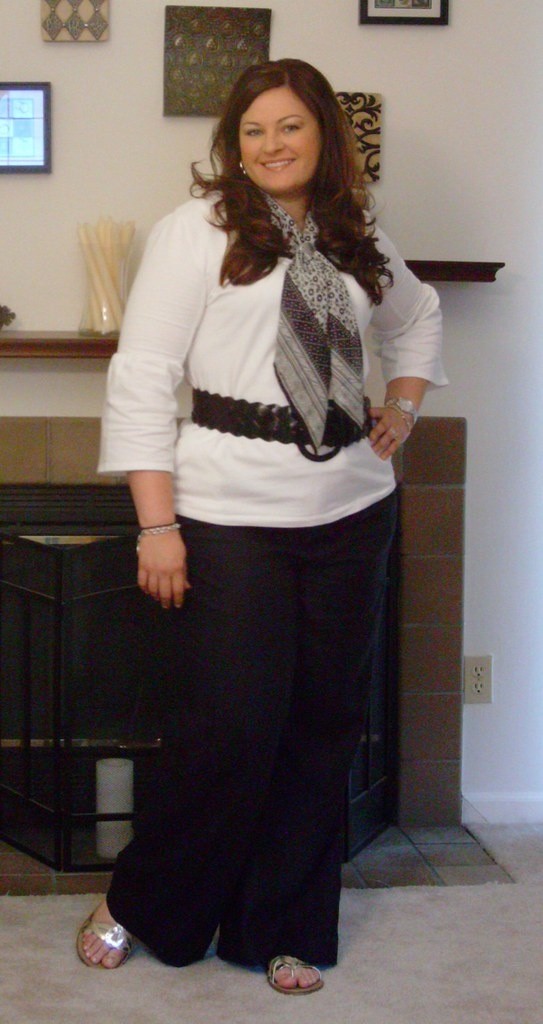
[464,654,493,704]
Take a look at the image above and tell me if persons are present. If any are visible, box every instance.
[79,60,446,992]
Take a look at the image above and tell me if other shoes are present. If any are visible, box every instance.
[268,955,324,994]
[76,898,141,970]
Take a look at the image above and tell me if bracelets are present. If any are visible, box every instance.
[385,405,411,433]
[140,522,181,536]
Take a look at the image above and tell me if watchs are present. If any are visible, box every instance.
[385,397,418,425]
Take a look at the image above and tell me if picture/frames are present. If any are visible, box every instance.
[0,81,52,175]
[358,0,448,26]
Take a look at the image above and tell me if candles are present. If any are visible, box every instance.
[74,217,136,337]
